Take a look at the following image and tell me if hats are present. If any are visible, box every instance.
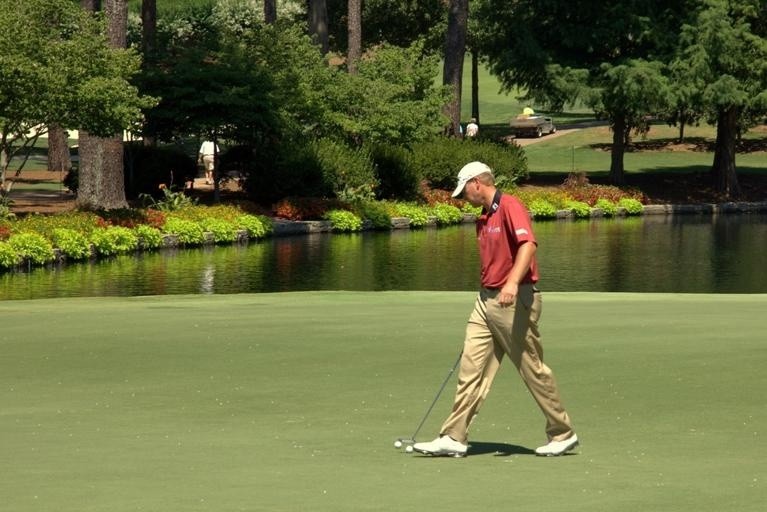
[450,161,491,200]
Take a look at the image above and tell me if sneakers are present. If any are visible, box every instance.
[413,435,469,458]
[535,433,578,457]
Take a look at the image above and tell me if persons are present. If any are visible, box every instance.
[460,123,463,139]
[523,104,534,114]
[198,134,220,185]
[464,118,478,137]
[414,161,580,457]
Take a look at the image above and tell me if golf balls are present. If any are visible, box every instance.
[394,441,402,448]
[406,446,413,452]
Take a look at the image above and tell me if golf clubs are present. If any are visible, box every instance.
[396,350,465,444]
